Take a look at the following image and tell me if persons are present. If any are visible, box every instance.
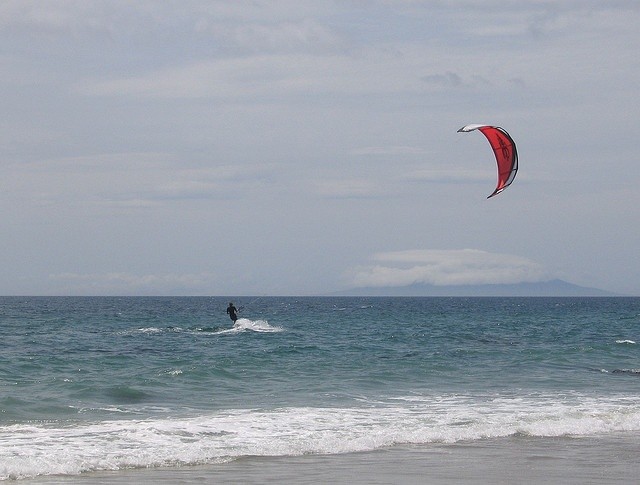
[227,302,239,324]
